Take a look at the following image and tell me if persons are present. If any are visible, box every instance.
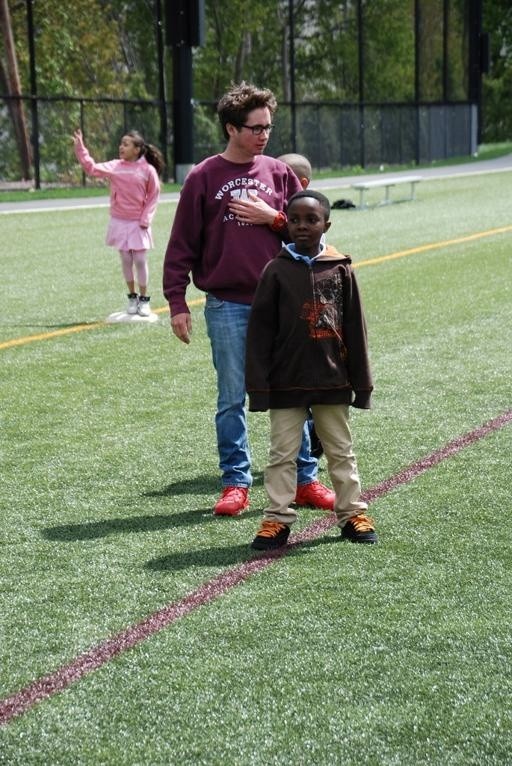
[162,75,337,517]
[70,126,168,318]
[242,190,380,552]
[271,152,328,473]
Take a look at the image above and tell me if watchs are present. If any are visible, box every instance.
[270,211,287,232]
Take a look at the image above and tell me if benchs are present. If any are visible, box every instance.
[352,175,423,211]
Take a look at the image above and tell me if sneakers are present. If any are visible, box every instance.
[216,487,248,515]
[127,293,151,316]
[295,480,335,510]
[252,521,289,549]
[341,516,377,543]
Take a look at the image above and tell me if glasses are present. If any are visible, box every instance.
[241,124,275,135]
